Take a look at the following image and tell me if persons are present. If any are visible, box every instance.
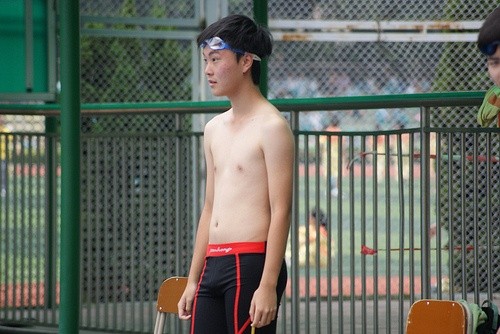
[178,15,295,334]
[284,114,343,266]
[478,5,500,334]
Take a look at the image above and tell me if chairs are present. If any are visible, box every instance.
[151,277,189,334]
[405,299,473,334]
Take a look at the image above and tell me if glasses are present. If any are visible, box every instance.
[477,39,500,56]
[198,36,262,62]
